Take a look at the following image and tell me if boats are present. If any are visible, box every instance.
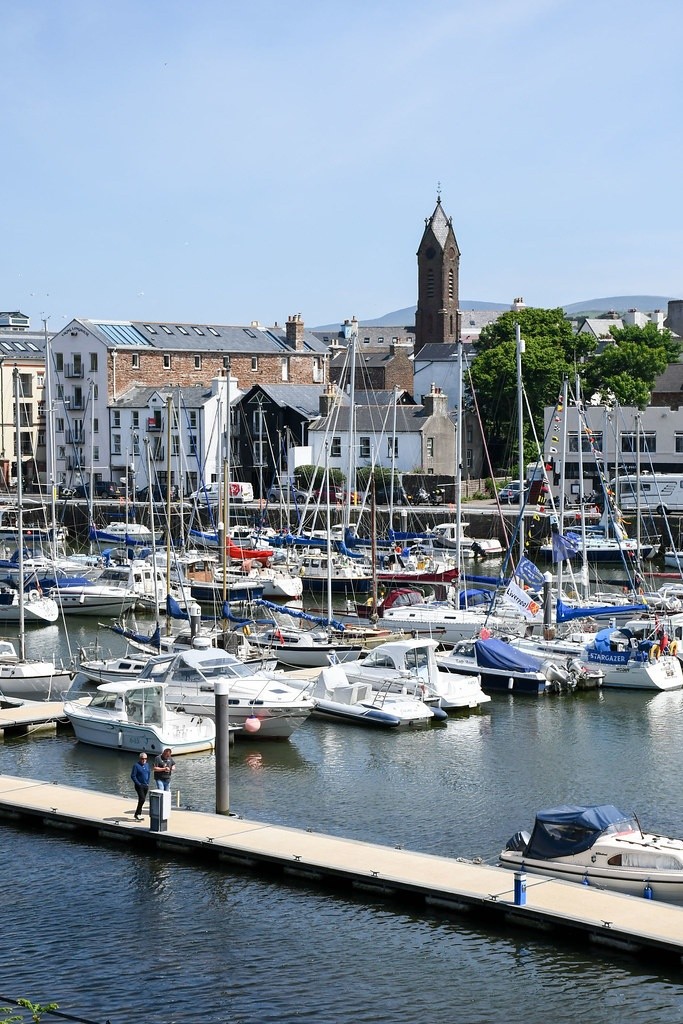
[499,803,683,904]
[58,676,216,756]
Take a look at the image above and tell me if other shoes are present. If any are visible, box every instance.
[134,814,144,820]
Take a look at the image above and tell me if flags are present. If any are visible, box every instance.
[497,532,581,620]
[520,394,647,606]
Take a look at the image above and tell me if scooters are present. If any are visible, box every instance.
[547,492,572,510]
[413,487,445,506]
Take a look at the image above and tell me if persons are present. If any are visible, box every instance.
[153,748,177,791]
[131,752,150,820]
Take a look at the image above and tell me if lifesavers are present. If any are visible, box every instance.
[648,644,660,658]
[28,589,41,602]
[669,640,679,656]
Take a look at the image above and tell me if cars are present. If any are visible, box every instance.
[267,483,312,504]
[313,485,362,504]
[73,480,122,499]
[498,483,529,504]
[367,485,413,506]
[128,483,180,502]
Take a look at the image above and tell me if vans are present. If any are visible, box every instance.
[188,481,254,505]
[609,470,683,516]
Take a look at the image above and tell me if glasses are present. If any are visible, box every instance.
[140,758,147,760]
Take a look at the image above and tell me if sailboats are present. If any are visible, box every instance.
[0,318,683,740]
